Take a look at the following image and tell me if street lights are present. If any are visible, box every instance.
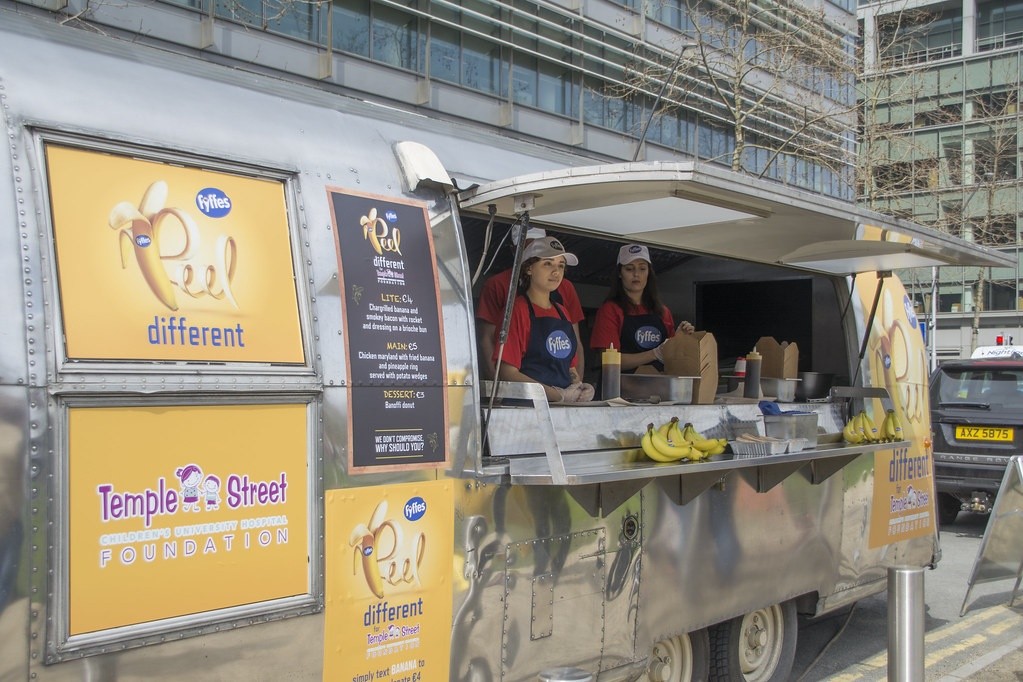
[929,171,996,371]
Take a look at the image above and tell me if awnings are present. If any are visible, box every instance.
[456,159,1017,403]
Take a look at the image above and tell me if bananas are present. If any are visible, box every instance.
[843,411,904,442]
[871,289,911,431]
[109,180,201,310]
[641,418,728,460]
[348,499,403,599]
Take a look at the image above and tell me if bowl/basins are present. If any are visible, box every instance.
[796,372,835,399]
[721,375,803,402]
[726,413,820,454]
[620,374,701,404]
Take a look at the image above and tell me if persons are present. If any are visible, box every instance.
[493,236,595,402]
[476,225,585,382]
[590,244,694,373]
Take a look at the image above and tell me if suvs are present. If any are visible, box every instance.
[930,345,1022,526]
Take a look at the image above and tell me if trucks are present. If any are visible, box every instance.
[1,29,1017,681]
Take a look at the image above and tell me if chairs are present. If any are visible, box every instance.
[982,374,1023,409]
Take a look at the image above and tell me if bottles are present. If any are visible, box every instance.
[602,342,621,401]
[744,346,762,399]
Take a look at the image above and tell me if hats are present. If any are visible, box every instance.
[521,235,579,267]
[617,243,652,266]
